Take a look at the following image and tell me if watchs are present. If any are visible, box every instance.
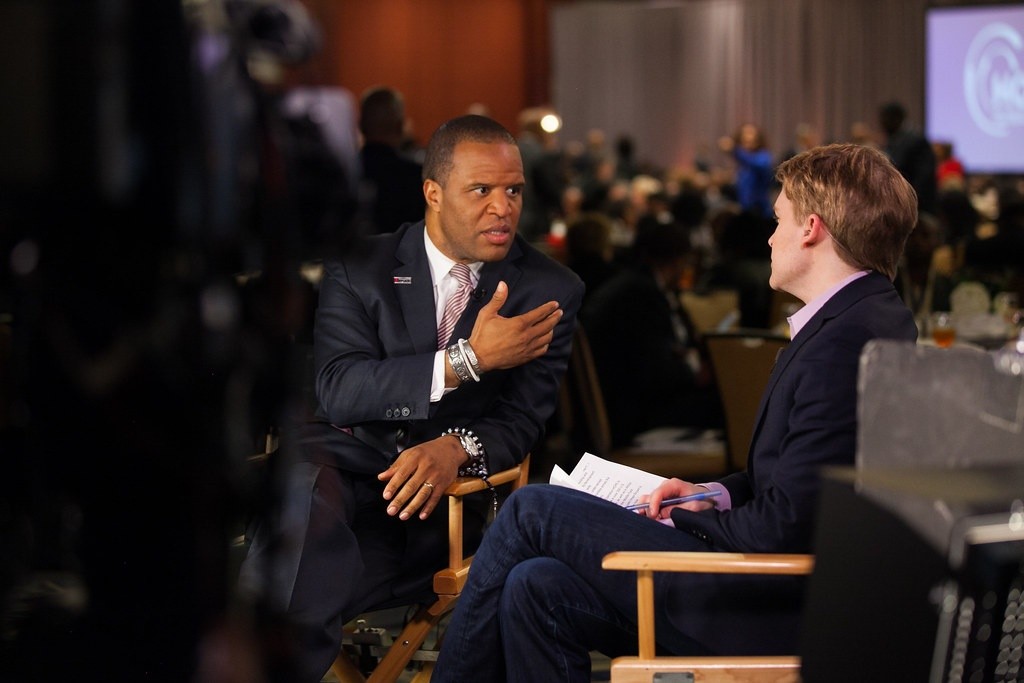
[443,433,480,469]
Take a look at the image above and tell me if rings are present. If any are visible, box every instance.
[423,482,435,488]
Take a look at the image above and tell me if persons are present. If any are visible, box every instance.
[257,114,586,683]
[234,1,1024,454]
[428,146,920,683]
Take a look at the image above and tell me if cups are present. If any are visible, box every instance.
[932,312,958,348]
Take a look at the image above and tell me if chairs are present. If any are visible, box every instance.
[307,246,976,683]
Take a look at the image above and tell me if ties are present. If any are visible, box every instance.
[437,263,475,351]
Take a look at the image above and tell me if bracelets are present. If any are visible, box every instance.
[458,338,480,382]
[441,427,498,519]
[448,343,471,382]
[463,340,483,375]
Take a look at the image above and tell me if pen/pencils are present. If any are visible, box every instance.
[625,491,723,511]
